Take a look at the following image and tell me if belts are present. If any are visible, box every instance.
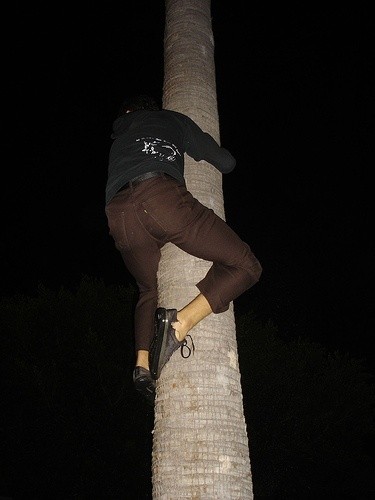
[114,170,161,195]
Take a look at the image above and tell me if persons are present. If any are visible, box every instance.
[104,94,263,402]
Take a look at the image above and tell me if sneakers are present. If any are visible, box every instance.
[132,366,156,407]
[148,307,194,380]
